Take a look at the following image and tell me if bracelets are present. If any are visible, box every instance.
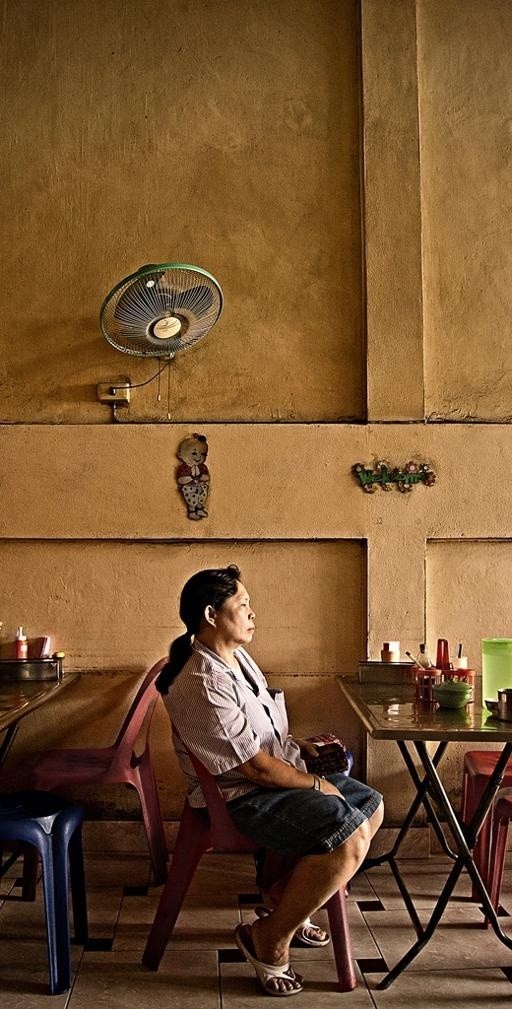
[288,734,293,738]
[313,773,321,791]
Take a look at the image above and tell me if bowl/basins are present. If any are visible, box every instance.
[432,678,472,708]
[436,706,471,728]
[483,715,502,729]
[484,698,497,715]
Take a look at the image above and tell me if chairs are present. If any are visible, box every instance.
[485,786,512,928]
[142,720,358,993]
[459,752,512,900]
[23,656,169,903]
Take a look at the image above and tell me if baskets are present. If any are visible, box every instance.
[411,639,475,704]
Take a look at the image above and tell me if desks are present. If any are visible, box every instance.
[334,673,512,991]
[2,673,80,764]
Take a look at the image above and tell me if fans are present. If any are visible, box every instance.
[99,263,223,362]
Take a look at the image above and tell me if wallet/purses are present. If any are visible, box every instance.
[301,744,348,776]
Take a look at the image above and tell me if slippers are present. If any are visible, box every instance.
[255,906,331,947]
[234,922,305,996]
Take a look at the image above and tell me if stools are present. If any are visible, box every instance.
[1,792,88,996]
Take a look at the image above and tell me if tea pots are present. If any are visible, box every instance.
[482,637,512,709]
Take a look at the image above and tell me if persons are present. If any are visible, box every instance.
[155,565,385,995]
[175,432,210,521]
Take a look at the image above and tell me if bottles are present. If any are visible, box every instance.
[413,644,434,667]
[13,627,28,659]
[54,652,65,678]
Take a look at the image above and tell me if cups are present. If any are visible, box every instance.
[497,688,511,721]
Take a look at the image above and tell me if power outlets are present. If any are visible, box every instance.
[97,382,130,402]
